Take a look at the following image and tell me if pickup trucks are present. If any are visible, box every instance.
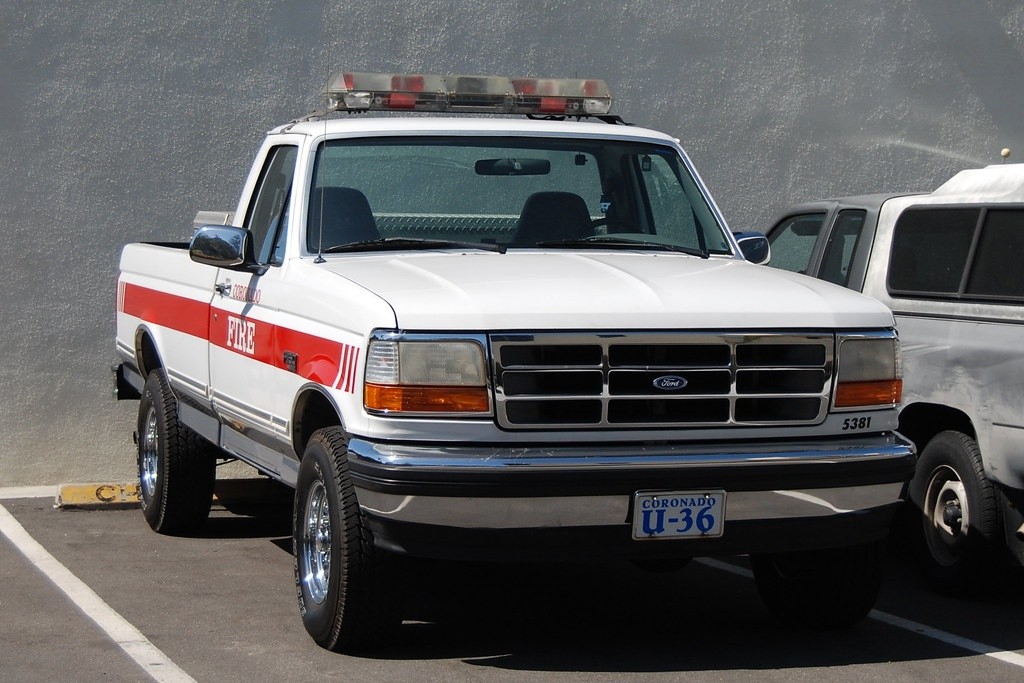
[766,158,1024,599]
[112,67,921,657]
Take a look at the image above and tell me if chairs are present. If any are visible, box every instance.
[513,191,595,244]
[310,187,381,249]
